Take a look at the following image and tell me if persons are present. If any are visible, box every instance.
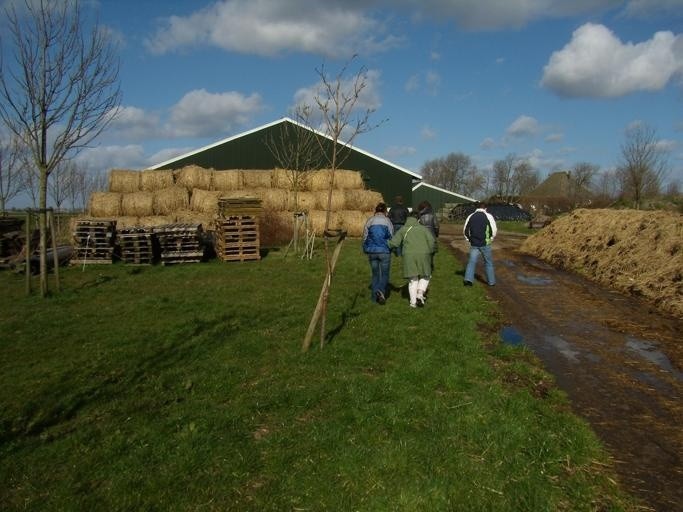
[362,194,438,308]
[463,201,498,288]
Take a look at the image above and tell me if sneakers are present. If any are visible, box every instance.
[375,290,386,305]
[463,279,473,287]
[416,295,425,307]
